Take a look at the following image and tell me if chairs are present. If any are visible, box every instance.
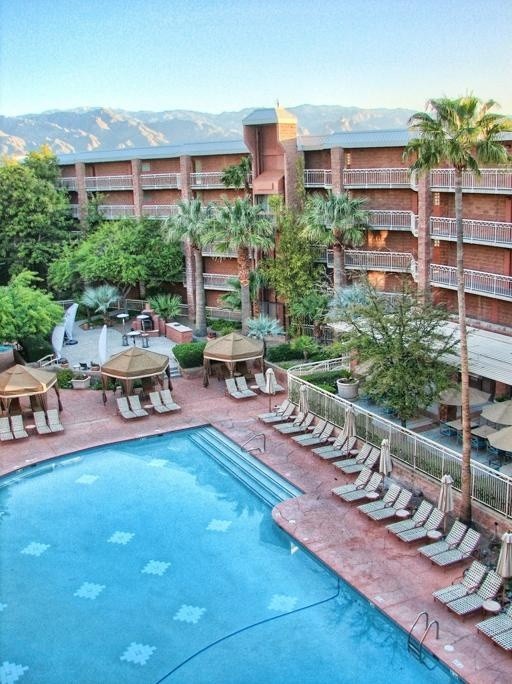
[0,408,64,441]
[116,388,184,421]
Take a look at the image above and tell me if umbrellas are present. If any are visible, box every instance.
[343,403,356,456]
[298,382,309,433]
[436,474,454,530]
[378,437,392,489]
[479,396,512,426]
[436,380,491,423]
[264,367,279,412]
[486,424,511,452]
[494,528,512,605]
